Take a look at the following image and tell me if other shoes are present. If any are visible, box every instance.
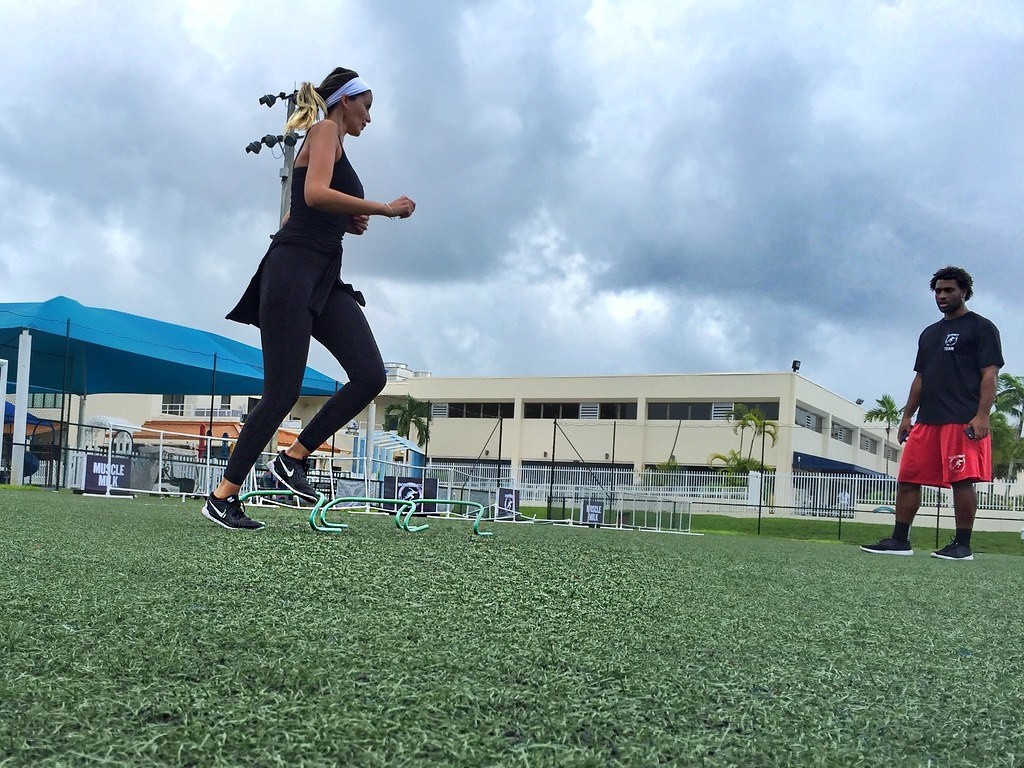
[930,535,974,561]
[859,537,914,555]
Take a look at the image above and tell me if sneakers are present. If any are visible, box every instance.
[266,450,319,504]
[201,492,266,531]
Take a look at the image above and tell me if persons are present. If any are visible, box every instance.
[859,266,1005,561]
[199,67,416,530]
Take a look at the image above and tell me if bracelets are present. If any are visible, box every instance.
[385,202,395,221]
